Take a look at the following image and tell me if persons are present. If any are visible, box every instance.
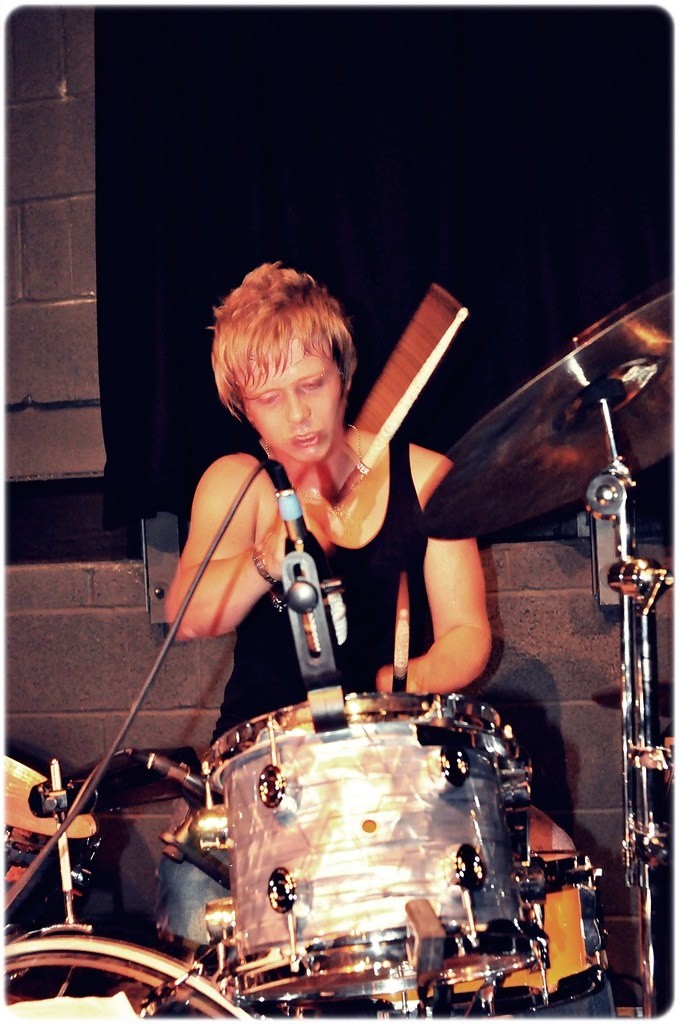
[166,264,494,762]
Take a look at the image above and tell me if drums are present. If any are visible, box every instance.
[431,848,606,1016]
[4,752,102,926]
[205,691,543,1001]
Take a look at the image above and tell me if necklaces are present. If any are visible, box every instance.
[264,423,363,520]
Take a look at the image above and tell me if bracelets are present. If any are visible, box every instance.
[251,538,283,587]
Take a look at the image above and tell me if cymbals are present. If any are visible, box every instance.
[418,276,672,541]
[591,664,673,719]
